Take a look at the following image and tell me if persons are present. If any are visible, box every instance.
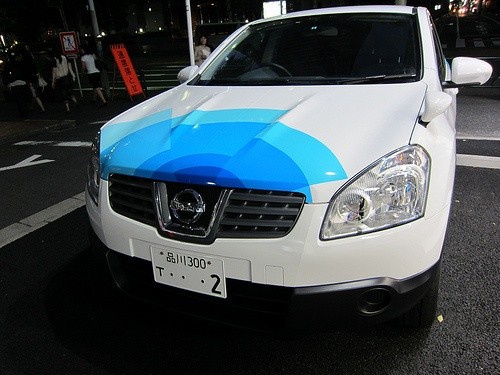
[80,43,109,107]
[50,48,78,113]
[194,34,211,68]
[12,48,46,115]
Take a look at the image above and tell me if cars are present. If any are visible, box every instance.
[86,4,494,342]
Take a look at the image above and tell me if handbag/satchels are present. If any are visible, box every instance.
[37,77,47,88]
